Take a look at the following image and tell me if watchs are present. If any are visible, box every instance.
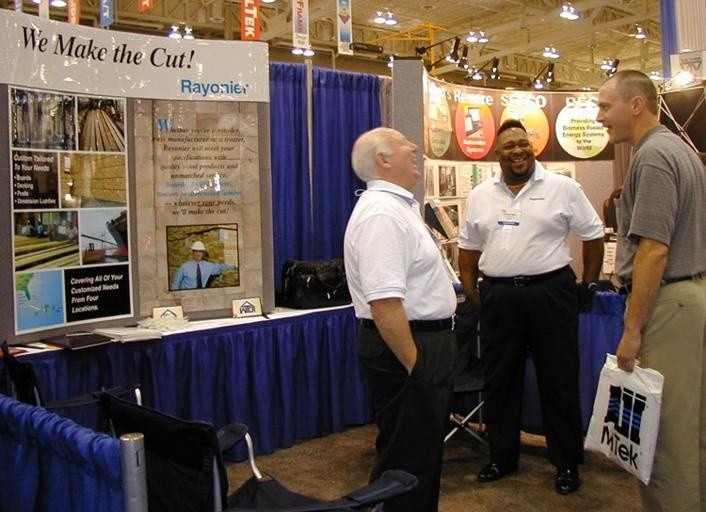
[581,280,598,293]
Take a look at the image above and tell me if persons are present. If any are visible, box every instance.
[595,68,705,511]
[13,214,77,242]
[457,120,605,497]
[171,240,238,291]
[341,125,456,511]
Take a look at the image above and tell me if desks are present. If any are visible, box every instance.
[1,305,373,462]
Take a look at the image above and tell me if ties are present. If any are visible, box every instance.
[197,264,202,288]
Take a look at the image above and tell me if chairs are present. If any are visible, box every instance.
[438,311,487,457]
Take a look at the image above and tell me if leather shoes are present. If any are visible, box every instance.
[478,459,518,483]
[555,466,580,495]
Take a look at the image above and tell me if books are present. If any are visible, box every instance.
[420,198,458,239]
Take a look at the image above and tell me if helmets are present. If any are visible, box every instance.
[191,241,206,251]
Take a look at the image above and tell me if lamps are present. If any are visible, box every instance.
[414,34,555,86]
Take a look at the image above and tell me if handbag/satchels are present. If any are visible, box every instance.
[282,259,353,309]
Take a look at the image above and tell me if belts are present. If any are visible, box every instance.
[361,315,461,333]
[618,272,705,297]
[482,265,574,287]
